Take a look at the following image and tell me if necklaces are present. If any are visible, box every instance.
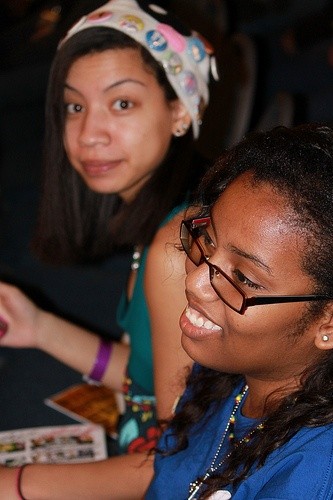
[187,381,301,500]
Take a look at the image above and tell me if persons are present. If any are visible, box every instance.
[144,122,333,500]
[0,0,218,500]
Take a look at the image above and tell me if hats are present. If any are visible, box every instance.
[57,0,220,140]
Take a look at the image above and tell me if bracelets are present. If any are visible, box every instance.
[17,463,30,500]
[90,338,111,382]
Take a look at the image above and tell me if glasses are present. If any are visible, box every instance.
[178,215,332,315]
